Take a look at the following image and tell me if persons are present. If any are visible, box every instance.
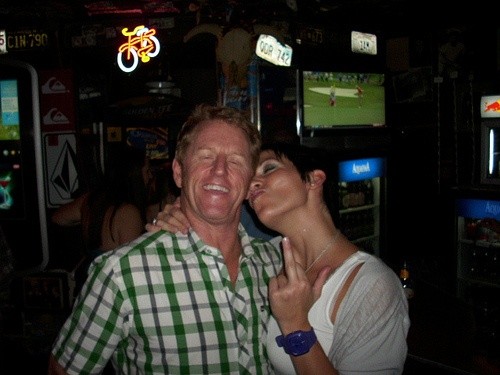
[145,141,410,375]
[53,135,173,255]
[49,106,282,375]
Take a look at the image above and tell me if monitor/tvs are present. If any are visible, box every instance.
[480,120,500,184]
[0,60,50,277]
[295,62,392,148]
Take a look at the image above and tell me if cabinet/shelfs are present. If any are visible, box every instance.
[455,196,500,324]
[324,155,389,266]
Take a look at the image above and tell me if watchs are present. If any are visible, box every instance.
[276,328,317,356]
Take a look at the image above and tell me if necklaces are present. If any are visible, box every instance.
[303,229,341,274]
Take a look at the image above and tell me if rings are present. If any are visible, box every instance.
[152,218,158,226]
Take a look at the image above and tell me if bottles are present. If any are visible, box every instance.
[340,178,375,210]
[400,259,414,310]
